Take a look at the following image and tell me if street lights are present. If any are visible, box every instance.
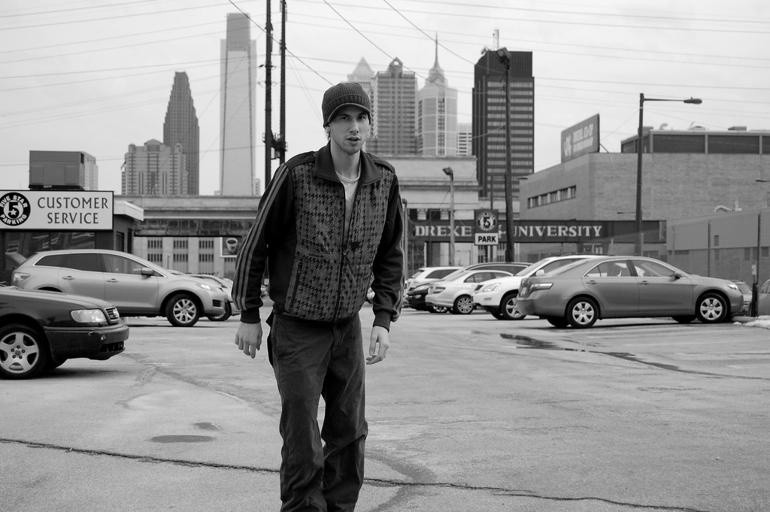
[635,93,702,253]
[500,47,515,262]
[442,167,455,265]
[401,198,409,280]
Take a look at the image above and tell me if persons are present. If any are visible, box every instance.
[230,81,406,511]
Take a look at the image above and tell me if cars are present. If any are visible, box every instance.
[0,248,267,382]
[733,278,768,315]
[366,254,646,323]
[515,256,745,330]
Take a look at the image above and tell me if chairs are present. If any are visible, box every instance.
[606,265,622,276]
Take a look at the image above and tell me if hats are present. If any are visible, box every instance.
[322,82,371,127]
